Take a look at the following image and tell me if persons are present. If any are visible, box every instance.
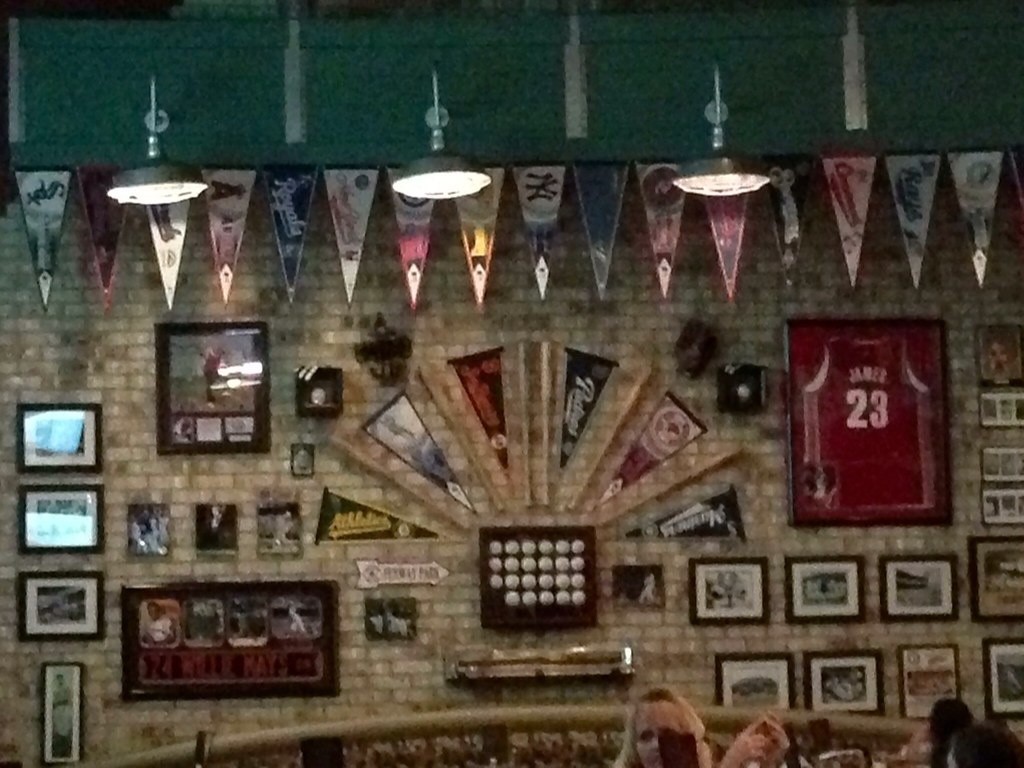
[612,686,789,768]
[52,674,70,755]
[929,699,1024,768]
[257,509,294,549]
[638,572,656,605]
[287,601,306,634]
[127,512,168,555]
[143,602,175,646]
[202,342,222,406]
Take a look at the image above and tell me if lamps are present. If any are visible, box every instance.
[392,69,491,200]
[106,74,209,205]
[672,63,771,196]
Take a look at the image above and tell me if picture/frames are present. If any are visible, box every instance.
[784,554,863,624]
[39,661,84,767]
[878,554,957,622]
[896,643,961,721]
[688,557,770,624]
[802,648,884,716]
[18,484,104,554]
[18,570,104,640]
[154,322,272,455]
[715,652,797,710]
[16,403,103,473]
[981,637,1024,722]
[966,535,1024,622]
[121,579,341,699]
